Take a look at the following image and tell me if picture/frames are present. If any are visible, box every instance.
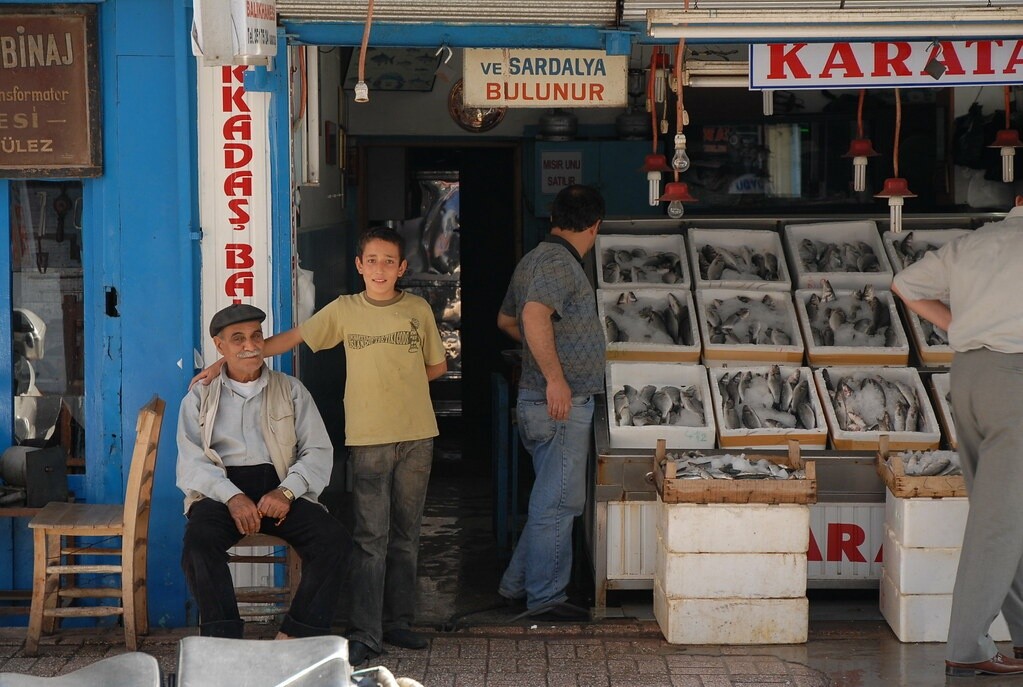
[448,77,508,133]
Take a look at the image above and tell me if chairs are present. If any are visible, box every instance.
[168,634,425,687]
[0,651,165,687]
[229,534,302,617]
[25,394,167,650]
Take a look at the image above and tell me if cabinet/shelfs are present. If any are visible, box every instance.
[523,138,668,285]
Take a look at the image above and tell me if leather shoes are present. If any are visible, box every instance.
[945,652,1023,676]
[1013,647,1023,659]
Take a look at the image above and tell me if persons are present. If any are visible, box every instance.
[890,182,1023,676]
[187,228,449,664]
[497,185,606,622]
[175,304,350,639]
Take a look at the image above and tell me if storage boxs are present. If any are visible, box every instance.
[650,437,818,644]
[875,434,1013,642]
[594,218,975,454]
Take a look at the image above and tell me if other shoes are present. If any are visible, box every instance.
[527,595,592,623]
[348,640,378,666]
[382,629,428,649]
[494,592,528,615]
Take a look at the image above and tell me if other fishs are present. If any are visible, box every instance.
[602,247,683,285]
[719,364,817,429]
[886,449,962,478]
[697,244,781,283]
[799,237,881,274]
[707,295,790,346]
[892,232,936,268]
[822,369,921,431]
[807,279,897,346]
[614,385,705,426]
[660,451,806,480]
[919,314,949,346]
[604,291,691,347]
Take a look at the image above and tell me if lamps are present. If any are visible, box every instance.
[645,8,1023,36]
[682,59,752,89]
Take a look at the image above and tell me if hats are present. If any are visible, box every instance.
[210,304,266,338]
